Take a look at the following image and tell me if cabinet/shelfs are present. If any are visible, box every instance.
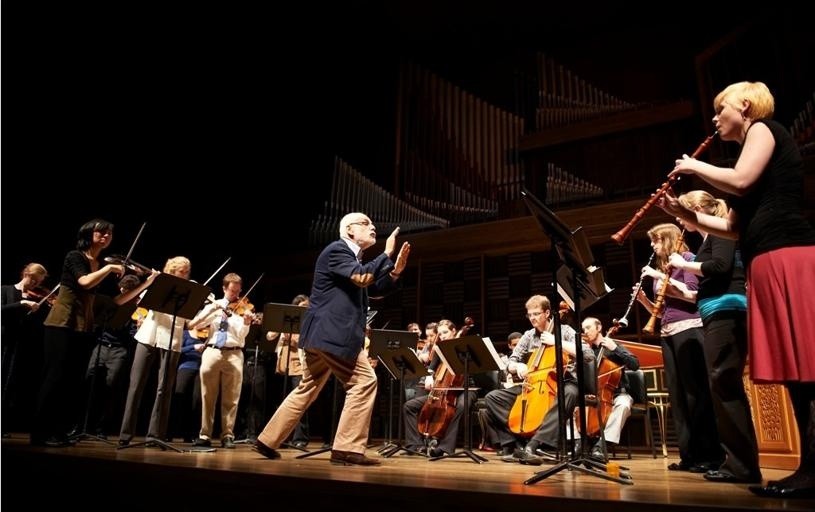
[742,354,801,471]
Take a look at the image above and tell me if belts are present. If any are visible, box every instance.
[100,341,126,347]
[207,344,243,350]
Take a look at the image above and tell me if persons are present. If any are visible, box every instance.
[668,80,815,501]
[250,212,410,466]
[27,218,160,448]
[186,272,256,448]
[400,319,478,459]
[417,322,439,389]
[675,188,765,485]
[266,293,312,450]
[1,262,53,440]
[75,274,143,441]
[570,317,640,463]
[163,324,214,443]
[478,332,527,451]
[484,294,596,466]
[404,322,429,401]
[115,255,228,450]
[237,324,266,445]
[630,223,720,473]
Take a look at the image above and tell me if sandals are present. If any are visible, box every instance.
[765,480,815,498]
[705,470,762,483]
[749,482,773,496]
[689,464,711,472]
[667,462,687,470]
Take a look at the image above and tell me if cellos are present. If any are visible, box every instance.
[508,301,569,437]
[417,316,474,440]
[576,318,623,436]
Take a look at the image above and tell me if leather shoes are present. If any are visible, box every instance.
[513,448,527,457]
[430,447,443,456]
[498,445,511,455]
[330,451,382,468]
[221,438,235,449]
[145,440,158,446]
[252,441,280,460]
[567,440,584,455]
[195,438,211,446]
[590,447,605,458]
[119,438,129,445]
[410,447,427,455]
[295,441,306,448]
[96,432,107,439]
[520,448,542,464]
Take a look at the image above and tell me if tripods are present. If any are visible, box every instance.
[60,295,117,446]
[429,375,489,464]
[276,325,312,453]
[116,315,184,454]
[384,369,428,458]
[234,340,270,445]
[376,381,400,453]
[296,377,357,459]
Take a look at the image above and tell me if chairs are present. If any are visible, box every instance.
[556,359,609,464]
[612,369,658,460]
[472,369,501,451]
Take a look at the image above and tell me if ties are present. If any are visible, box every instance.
[216,302,232,348]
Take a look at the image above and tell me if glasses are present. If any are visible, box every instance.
[349,219,372,226]
[526,312,544,318]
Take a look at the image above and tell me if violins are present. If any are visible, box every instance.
[104,254,152,276]
[131,306,148,322]
[227,295,258,324]
[196,326,210,340]
[28,284,58,307]
